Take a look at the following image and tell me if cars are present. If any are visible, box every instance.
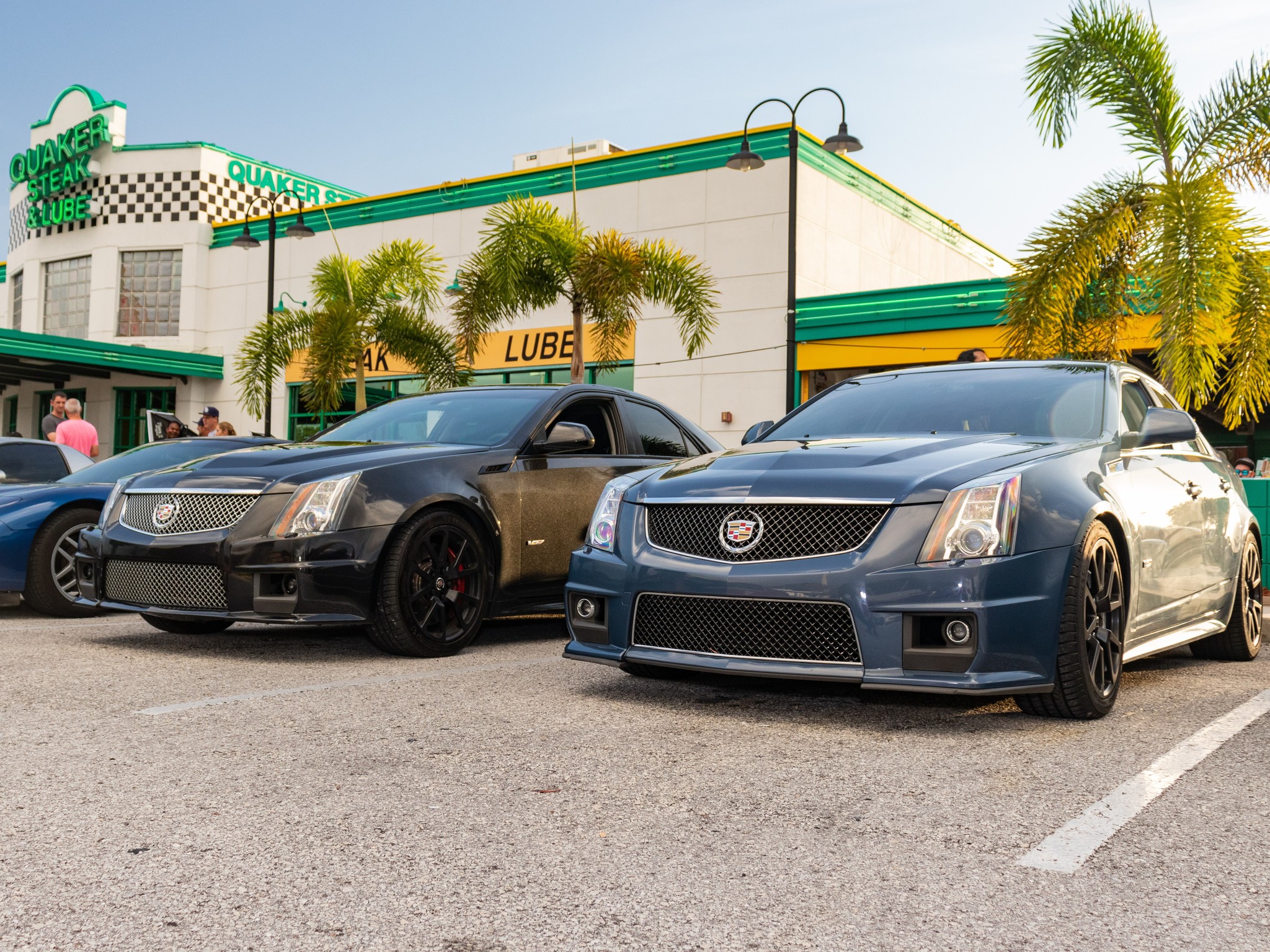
[564,359,1264,722]
[1,437,296,621]
[1,438,95,507]
[74,382,725,660]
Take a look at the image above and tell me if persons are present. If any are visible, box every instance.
[215,421,236,436]
[164,421,181,439]
[192,417,210,437]
[198,406,219,437]
[956,348,989,362]
[41,391,69,442]
[55,398,99,457]
[1235,457,1256,478]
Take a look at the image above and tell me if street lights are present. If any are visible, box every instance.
[727,88,864,421]
[231,189,316,437]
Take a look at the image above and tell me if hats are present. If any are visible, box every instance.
[192,417,205,426]
[197,406,219,417]
